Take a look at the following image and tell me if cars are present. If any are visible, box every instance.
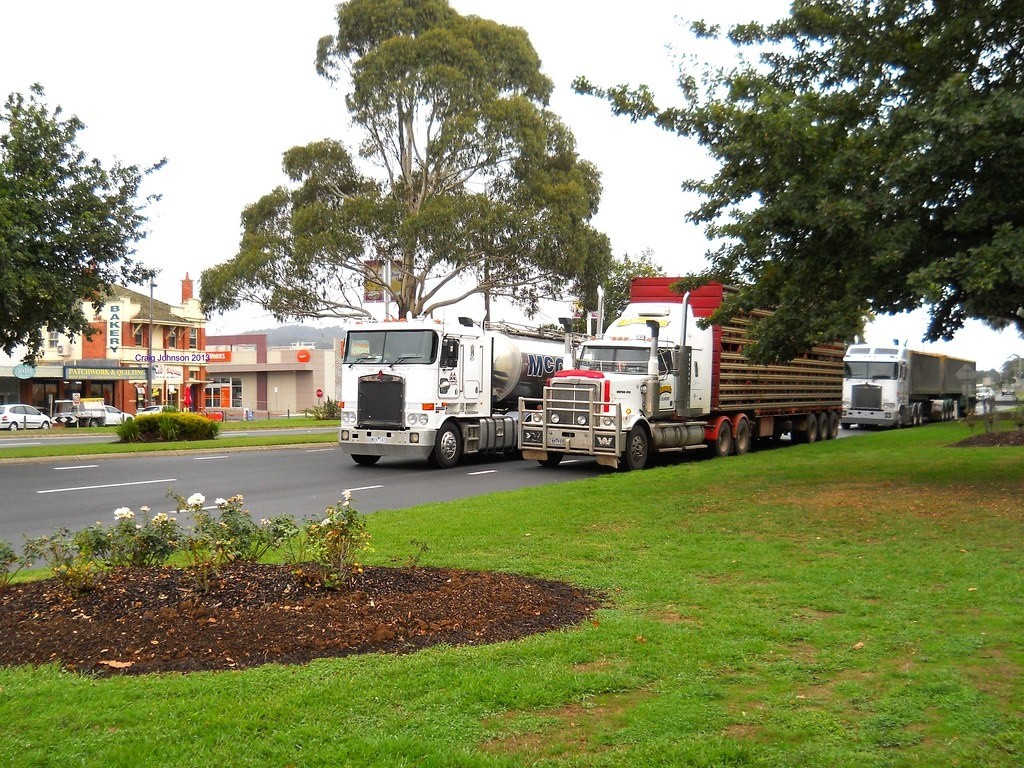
[105,405,163,426]
[1002,384,1016,396]
[976,386,996,400]
[0,404,50,431]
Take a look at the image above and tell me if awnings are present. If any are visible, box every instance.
[130,310,193,341]
[183,380,214,389]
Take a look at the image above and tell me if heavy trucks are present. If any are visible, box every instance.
[518,277,846,470]
[338,316,596,469]
[840,344,976,430]
